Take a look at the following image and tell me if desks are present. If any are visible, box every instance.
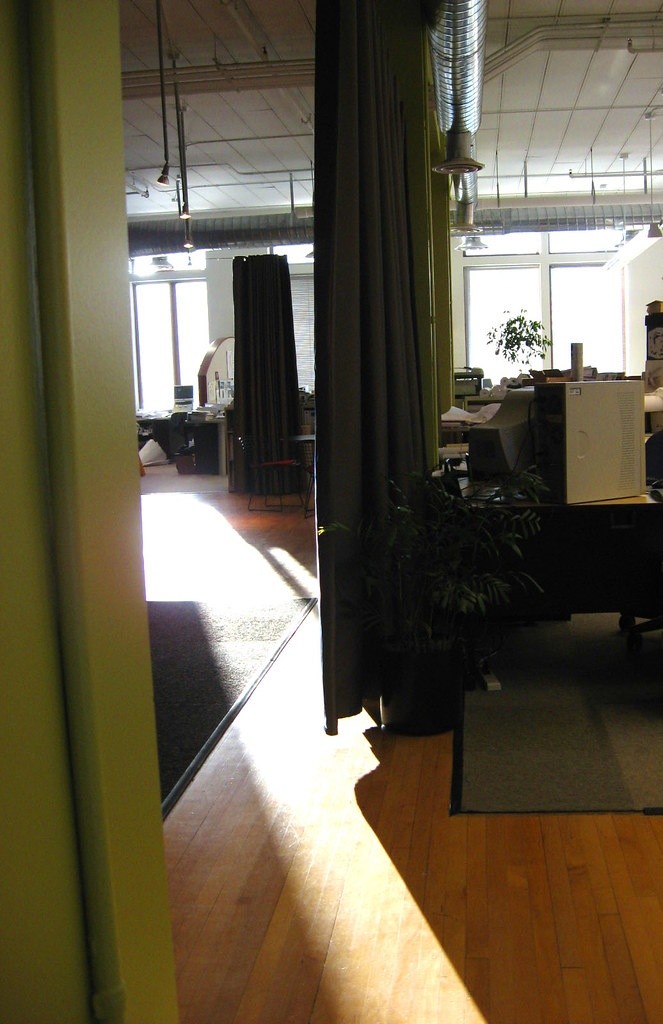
[281,434,315,442]
[473,494,663,622]
[136,415,228,475]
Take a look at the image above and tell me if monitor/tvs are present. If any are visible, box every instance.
[469,386,536,486]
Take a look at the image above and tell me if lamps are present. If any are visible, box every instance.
[179,203,191,219]
[183,234,194,249]
[598,112,663,275]
[157,162,169,186]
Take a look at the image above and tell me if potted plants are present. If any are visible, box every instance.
[317,465,549,736]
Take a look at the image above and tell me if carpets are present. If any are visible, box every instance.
[146,596,317,824]
[451,614,663,816]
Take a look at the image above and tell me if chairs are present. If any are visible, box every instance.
[235,433,304,512]
[304,465,314,518]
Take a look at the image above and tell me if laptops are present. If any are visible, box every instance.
[172,385,193,412]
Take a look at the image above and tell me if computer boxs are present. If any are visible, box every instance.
[534,379,645,505]
